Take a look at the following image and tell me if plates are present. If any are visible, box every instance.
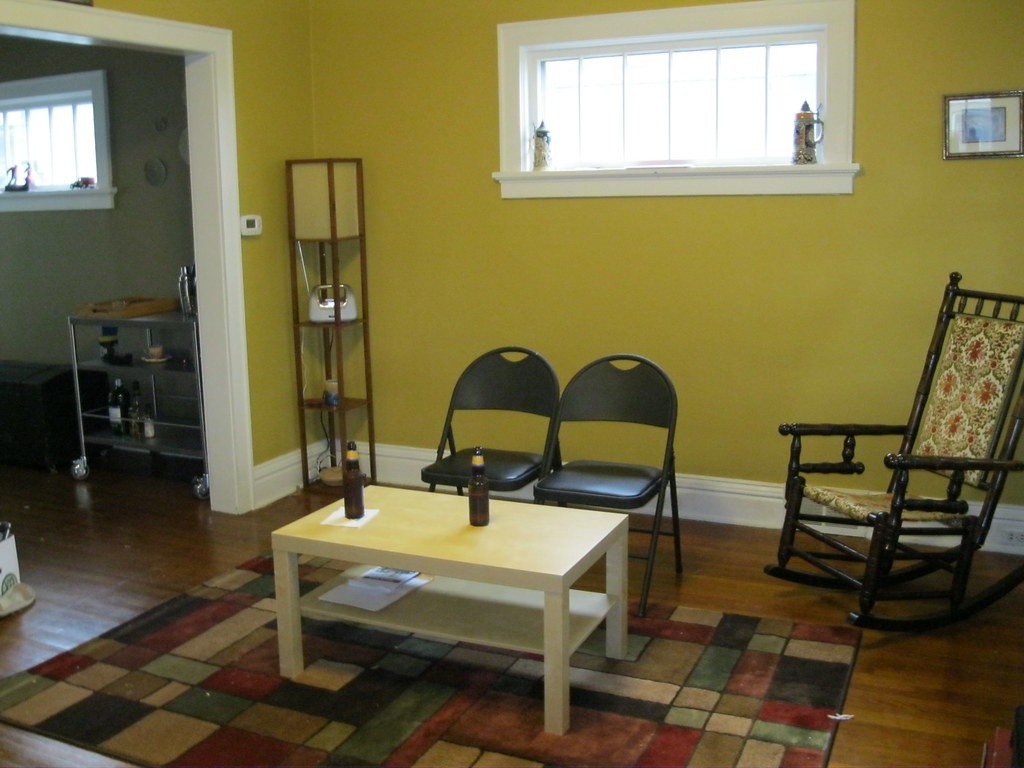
[141,354,172,362]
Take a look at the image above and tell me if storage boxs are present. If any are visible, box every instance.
[0,359,110,475]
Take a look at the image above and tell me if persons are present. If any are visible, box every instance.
[7,164,17,186]
[24,162,32,187]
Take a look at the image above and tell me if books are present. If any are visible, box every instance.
[347,567,420,593]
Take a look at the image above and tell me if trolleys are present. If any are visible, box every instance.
[66,307,210,500]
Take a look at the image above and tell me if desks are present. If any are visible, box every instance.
[265,483,642,739]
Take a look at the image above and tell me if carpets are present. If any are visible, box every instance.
[0,550,864,768]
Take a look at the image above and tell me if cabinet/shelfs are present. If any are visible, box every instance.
[65,297,208,488]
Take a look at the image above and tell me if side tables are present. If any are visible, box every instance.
[287,152,377,489]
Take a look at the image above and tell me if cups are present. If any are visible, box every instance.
[148,346,163,359]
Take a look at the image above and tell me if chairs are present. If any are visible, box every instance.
[522,346,684,615]
[417,348,562,498]
[761,269,1024,637]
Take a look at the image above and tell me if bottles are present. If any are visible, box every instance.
[342,441,364,520]
[468,447,490,527]
[178,265,196,315]
[107,378,149,436]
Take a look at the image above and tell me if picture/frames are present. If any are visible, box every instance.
[941,87,1024,162]
